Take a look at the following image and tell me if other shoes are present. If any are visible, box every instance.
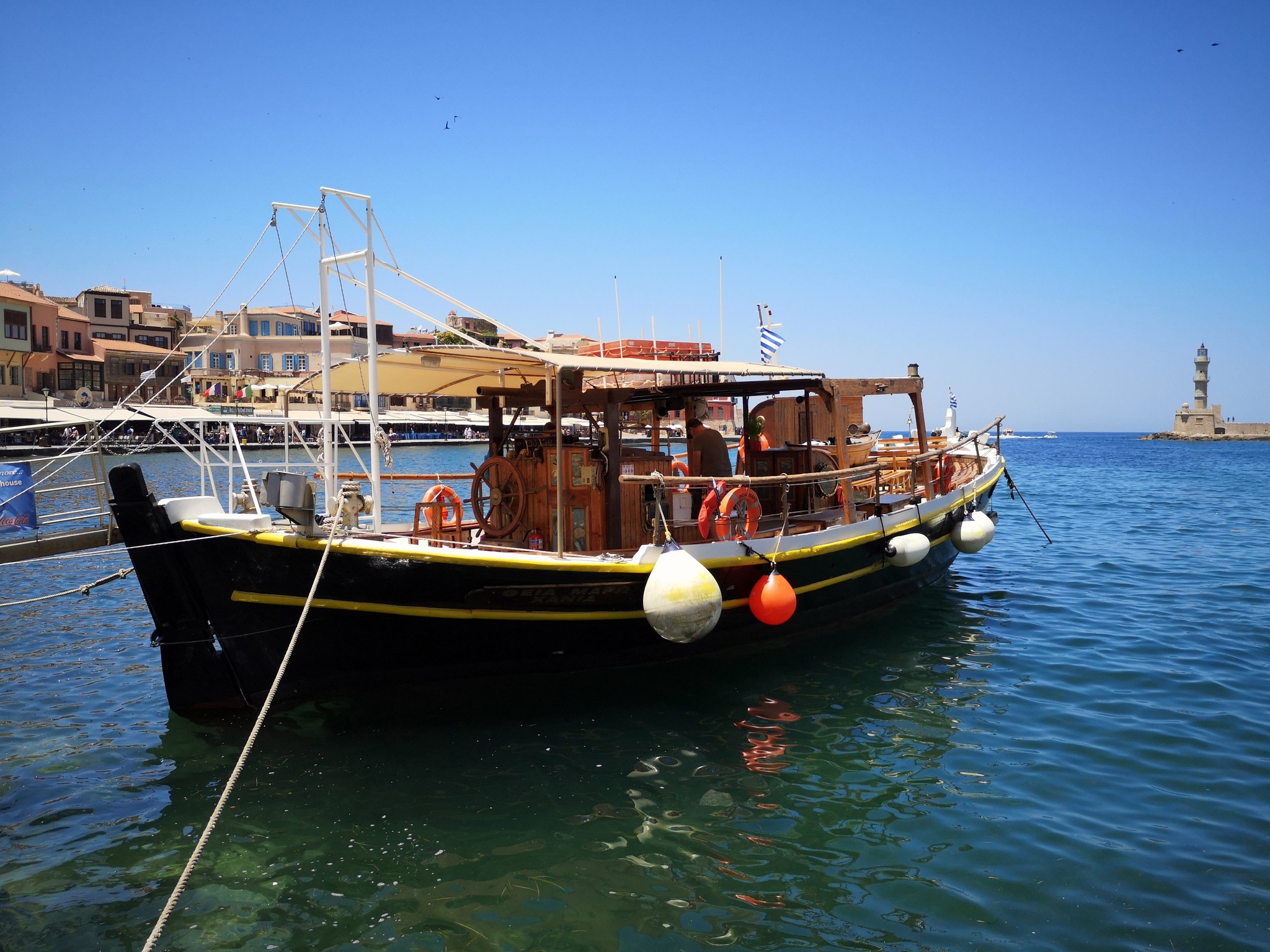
[192,444,193,446]
[466,439,467,440]
[65,444,68,446]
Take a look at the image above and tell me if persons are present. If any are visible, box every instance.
[464,427,472,439]
[393,425,489,438]
[351,428,370,436]
[685,418,735,542]
[735,427,742,436]
[625,415,627,421]
[128,426,136,445]
[561,427,572,435]
[618,422,623,431]
[161,425,317,445]
[44,426,79,446]
[389,428,392,434]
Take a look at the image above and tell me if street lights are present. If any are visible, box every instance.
[233,398,240,426]
[443,406,448,441]
[518,410,522,432]
[668,411,671,428]
[41,386,52,437]
[336,404,341,442]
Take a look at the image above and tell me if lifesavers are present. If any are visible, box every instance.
[932,455,954,495]
[738,433,769,463]
[422,485,463,527]
[672,461,689,492]
[716,487,760,542]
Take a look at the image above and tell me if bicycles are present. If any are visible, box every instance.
[292,435,301,443]
[260,434,270,445]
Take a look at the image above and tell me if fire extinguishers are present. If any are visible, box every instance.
[522,525,544,551]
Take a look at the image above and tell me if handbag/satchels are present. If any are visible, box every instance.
[270,435,274,438]
[66,436,72,441]
[69,428,71,434]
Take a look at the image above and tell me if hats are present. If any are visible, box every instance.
[469,427,471,429]
[477,431,479,433]
[466,428,468,429]
[685,418,701,439]
[243,426,246,429]
[258,427,261,429]
[72,427,76,429]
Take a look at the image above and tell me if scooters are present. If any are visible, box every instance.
[204,433,219,445]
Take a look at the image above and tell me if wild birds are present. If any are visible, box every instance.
[454,116,461,123]
[443,121,450,130]
[434,96,441,100]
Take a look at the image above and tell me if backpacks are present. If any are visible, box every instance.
[127,429,132,435]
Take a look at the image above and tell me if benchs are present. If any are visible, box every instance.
[787,509,867,530]
[854,494,921,519]
[754,519,796,538]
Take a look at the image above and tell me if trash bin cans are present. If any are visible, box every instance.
[340,437,345,443]
[444,434,447,441]
[38,436,48,447]
[242,439,248,445]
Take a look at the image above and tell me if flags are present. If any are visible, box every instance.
[203,382,221,397]
[949,390,957,408]
[237,385,253,399]
[759,323,786,363]
[140,369,156,380]
[180,374,191,383]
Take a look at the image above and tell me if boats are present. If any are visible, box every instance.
[1001,428,1014,437]
[1045,430,1056,438]
[104,184,1017,721]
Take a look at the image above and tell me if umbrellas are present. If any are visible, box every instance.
[645,424,683,431]
[240,383,299,394]
